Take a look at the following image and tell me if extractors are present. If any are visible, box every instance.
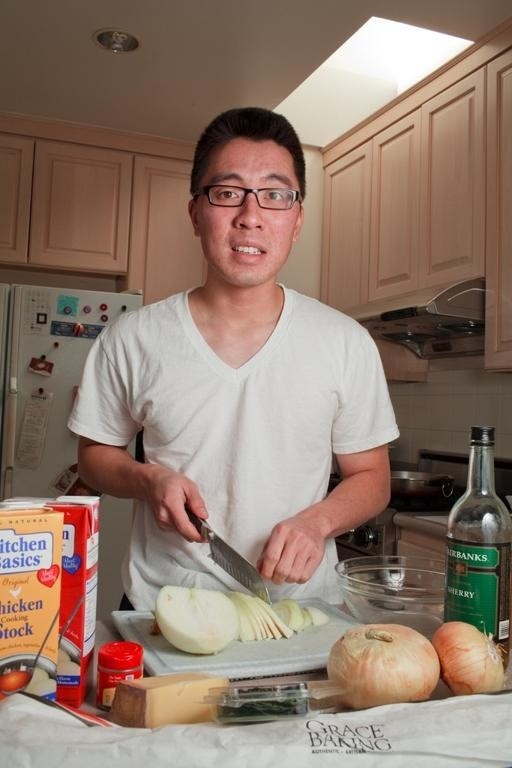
[344,277,485,359]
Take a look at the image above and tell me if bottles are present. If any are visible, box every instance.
[444,427,512,671]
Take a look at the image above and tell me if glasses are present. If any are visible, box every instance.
[193,185,302,210]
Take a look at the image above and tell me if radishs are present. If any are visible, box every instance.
[154,583,329,656]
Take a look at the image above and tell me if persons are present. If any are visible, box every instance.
[66,106,401,611]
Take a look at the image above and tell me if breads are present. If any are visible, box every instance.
[107,672,229,730]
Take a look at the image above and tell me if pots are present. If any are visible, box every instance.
[391,470,454,499]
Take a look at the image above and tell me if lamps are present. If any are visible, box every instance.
[91,28,141,54]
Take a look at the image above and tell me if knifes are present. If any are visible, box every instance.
[186,502,271,605]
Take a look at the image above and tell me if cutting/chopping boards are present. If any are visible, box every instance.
[110,597,366,679]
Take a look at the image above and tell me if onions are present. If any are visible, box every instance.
[431,621,507,698]
[327,622,441,711]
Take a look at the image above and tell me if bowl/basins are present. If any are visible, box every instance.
[336,555,447,622]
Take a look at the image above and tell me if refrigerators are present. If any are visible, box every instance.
[1,281,146,628]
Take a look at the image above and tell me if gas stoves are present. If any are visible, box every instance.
[330,448,512,557]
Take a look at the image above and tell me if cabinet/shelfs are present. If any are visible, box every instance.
[1,133,132,276]
[320,110,432,382]
[373,69,486,302]
[116,155,209,310]
[392,510,446,587]
[483,52,511,374]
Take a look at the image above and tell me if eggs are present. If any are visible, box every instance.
[0,670,32,692]
